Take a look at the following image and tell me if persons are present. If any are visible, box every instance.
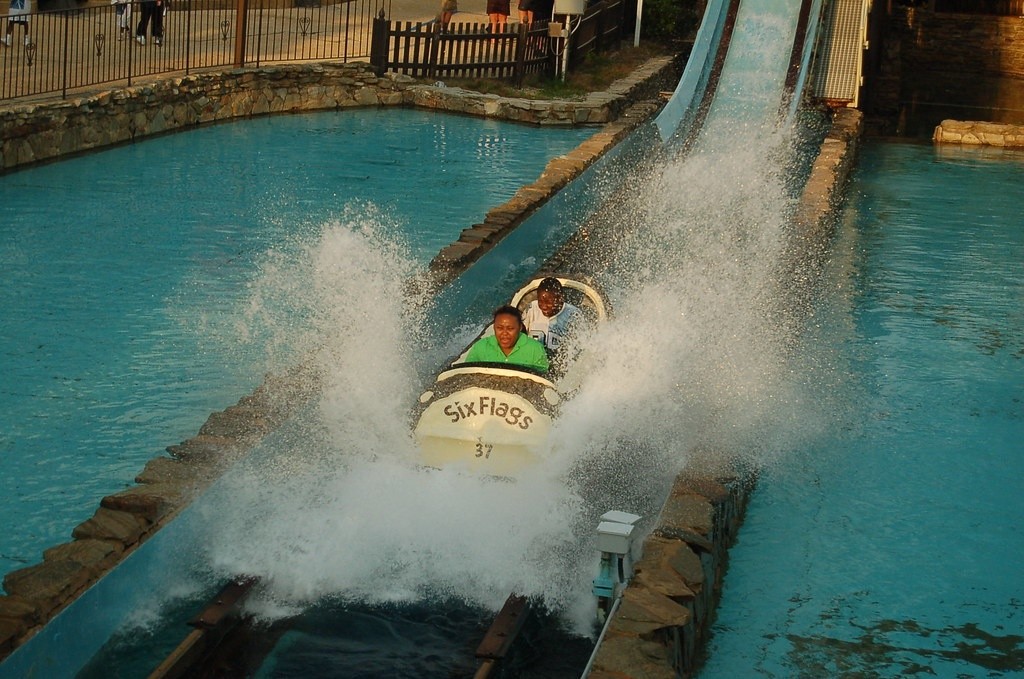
[518,0,534,45]
[465,305,549,368]
[0,0,32,47]
[486,0,510,44]
[110,0,168,45]
[521,277,584,351]
[439,0,458,31]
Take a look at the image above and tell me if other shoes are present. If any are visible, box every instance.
[126,30,129,38]
[118,33,124,41]
[154,37,159,44]
[1,34,11,46]
[137,35,145,45]
[23,35,28,45]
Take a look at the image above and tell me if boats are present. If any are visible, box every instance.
[412,265,615,476]
